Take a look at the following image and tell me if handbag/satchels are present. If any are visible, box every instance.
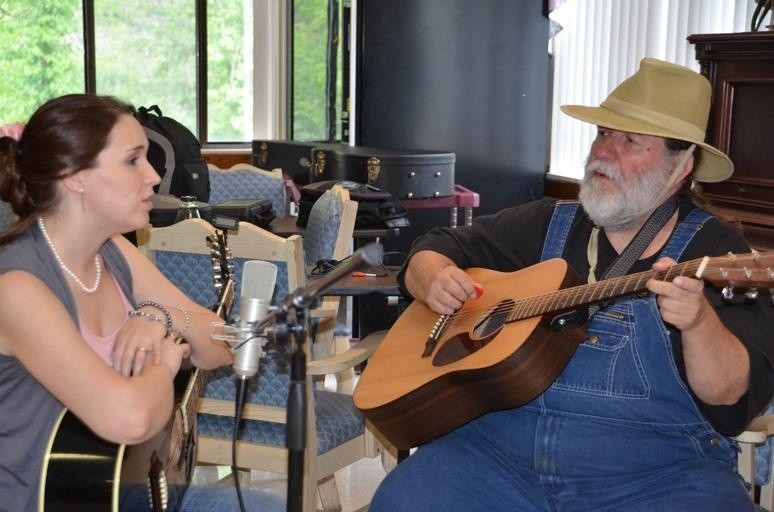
[136,105,209,225]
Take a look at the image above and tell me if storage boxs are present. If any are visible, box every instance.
[311,145,456,199]
[251,139,327,184]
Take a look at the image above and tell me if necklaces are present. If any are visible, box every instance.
[38,217,102,294]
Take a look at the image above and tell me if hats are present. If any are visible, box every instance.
[560,57,735,183]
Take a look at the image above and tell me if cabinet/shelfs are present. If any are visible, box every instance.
[686,30,774,253]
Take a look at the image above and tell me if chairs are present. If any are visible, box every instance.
[729,414,774,506]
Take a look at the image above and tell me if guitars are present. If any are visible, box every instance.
[353,249,774,451]
[38,229,236,512]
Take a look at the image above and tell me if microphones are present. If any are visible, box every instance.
[232,261,280,380]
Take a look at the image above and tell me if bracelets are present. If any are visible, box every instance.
[126,299,190,336]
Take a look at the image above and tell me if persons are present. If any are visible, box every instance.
[368,56,774,512]
[0,90,234,512]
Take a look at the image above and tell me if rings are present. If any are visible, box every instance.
[136,345,148,355]
